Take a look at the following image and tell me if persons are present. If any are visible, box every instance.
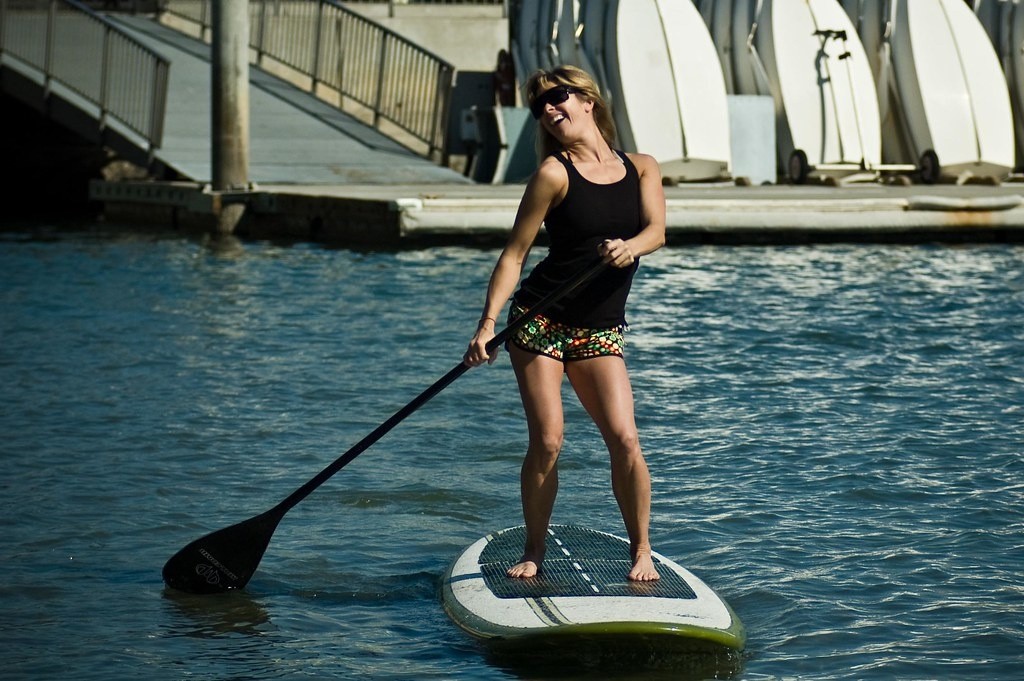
[464,65,666,581]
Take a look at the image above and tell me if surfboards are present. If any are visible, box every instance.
[441,523,744,652]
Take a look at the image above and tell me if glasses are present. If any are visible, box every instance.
[530,84,583,120]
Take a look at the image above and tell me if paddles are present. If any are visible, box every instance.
[162,253,609,599]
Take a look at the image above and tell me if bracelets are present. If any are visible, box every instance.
[479,317,496,323]
